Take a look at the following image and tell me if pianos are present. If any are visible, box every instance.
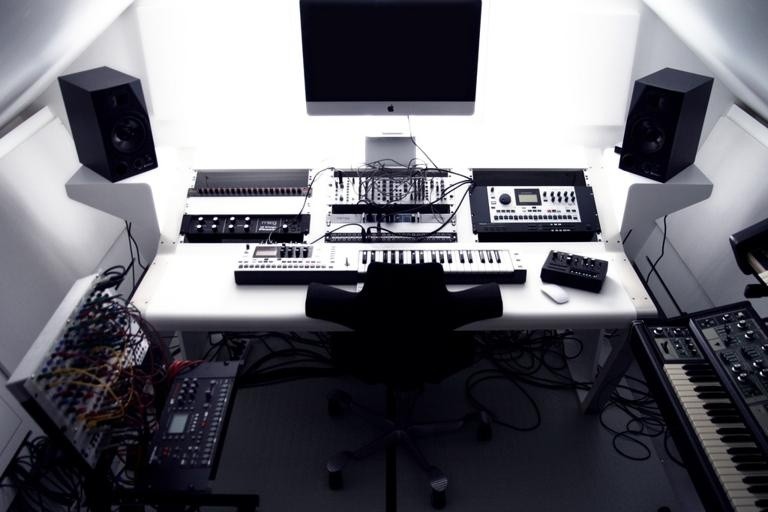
[235,240,526,287]
[580,301,766,509]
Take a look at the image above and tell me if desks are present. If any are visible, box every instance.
[65,141,714,436]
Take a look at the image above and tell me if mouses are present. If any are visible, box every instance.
[540,283,571,304]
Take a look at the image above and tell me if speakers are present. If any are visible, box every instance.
[57,65,159,184]
[615,67,715,184]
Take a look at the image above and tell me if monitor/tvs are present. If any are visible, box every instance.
[298,0,483,117]
